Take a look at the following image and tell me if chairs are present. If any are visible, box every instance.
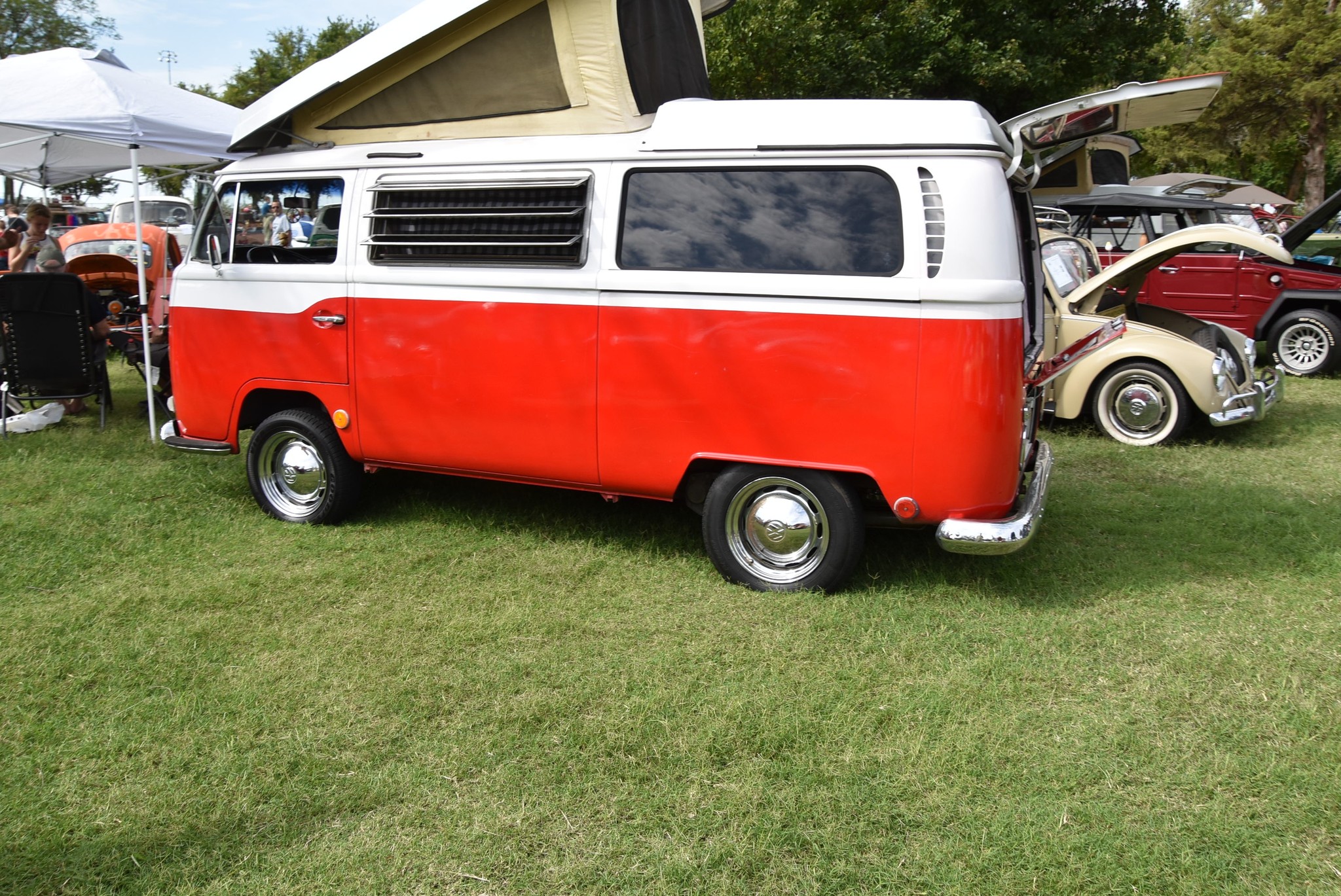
[0,272,114,438]
[110,312,172,421]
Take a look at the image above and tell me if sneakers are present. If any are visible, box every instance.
[138,394,168,408]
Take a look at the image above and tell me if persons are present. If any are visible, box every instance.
[6,203,62,273]
[0,220,8,271]
[242,204,252,225]
[271,199,293,249]
[35,244,111,415]
[288,214,298,223]
[262,196,273,217]
[138,312,173,407]
[6,206,28,233]
[299,209,312,221]
[262,208,275,245]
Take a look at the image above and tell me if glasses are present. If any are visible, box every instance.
[272,206,281,209]
[300,212,304,213]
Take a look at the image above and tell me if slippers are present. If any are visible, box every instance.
[59,403,86,416]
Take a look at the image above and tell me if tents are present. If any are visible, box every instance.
[0,47,258,446]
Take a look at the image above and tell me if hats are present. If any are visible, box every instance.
[314,209,319,213]
[36,245,66,270]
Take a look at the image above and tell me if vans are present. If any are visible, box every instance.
[161,68,1238,596]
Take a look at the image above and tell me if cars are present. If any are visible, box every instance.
[56,223,184,360]
[0,195,199,266]
[1023,222,1294,448]
[1055,191,1341,378]
